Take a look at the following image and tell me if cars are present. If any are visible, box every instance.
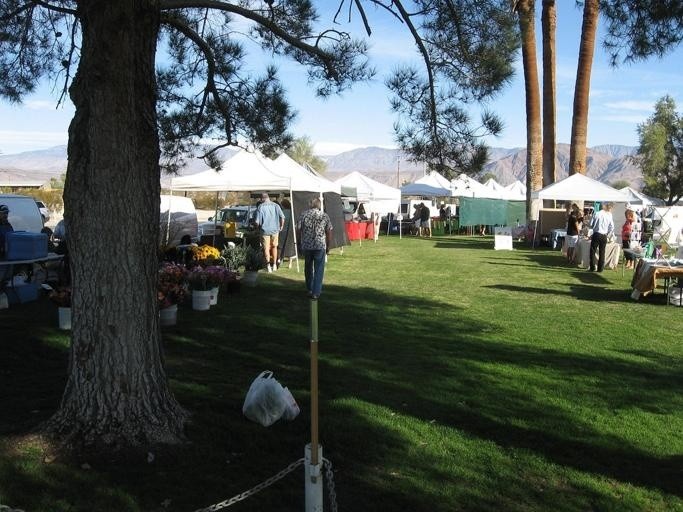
[201,206,260,237]
[50,192,200,254]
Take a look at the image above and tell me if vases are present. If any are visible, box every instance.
[58,306,72,330]
[159,303,178,326]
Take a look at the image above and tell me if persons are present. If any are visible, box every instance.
[565,203,615,274]
[0,204,15,291]
[440,205,446,218]
[39,226,68,288]
[296,195,333,300]
[412,202,421,237]
[620,209,635,269]
[251,191,286,273]
[53,216,66,239]
[479,225,485,235]
[418,203,433,238]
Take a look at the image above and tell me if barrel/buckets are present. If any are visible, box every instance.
[159,304,178,326]
[208,285,219,306]
[192,289,211,311]
[58,306,71,330]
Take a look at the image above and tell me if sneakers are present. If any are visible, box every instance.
[266,264,277,272]
[308,290,319,299]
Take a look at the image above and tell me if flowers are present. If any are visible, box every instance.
[48,284,72,308]
[155,243,236,311]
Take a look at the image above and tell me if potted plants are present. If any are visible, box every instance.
[226,241,270,287]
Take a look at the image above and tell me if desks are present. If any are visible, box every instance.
[549,228,620,272]
[622,248,683,306]
